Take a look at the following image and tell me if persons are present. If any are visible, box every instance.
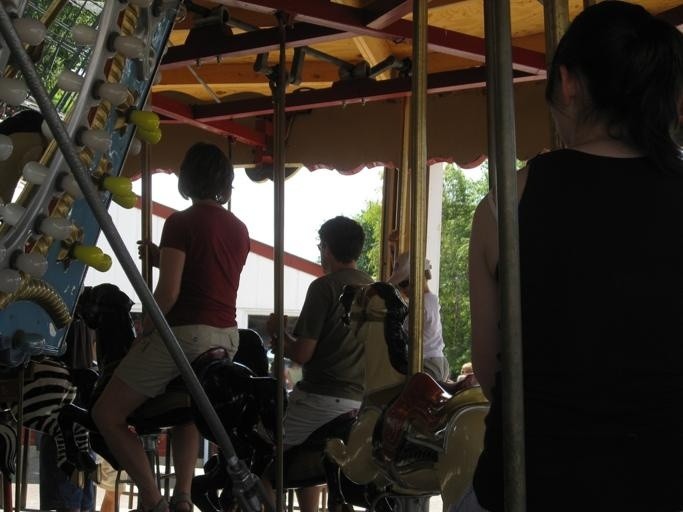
[92,143,250,512]
[266,216,376,511]
[469,0,683,511]
[385,251,451,385]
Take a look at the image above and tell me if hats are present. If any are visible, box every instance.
[387,250,431,284]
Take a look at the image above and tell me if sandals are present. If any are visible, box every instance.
[137,496,170,512]
[171,493,194,512]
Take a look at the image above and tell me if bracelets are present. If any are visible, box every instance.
[141,330,154,337]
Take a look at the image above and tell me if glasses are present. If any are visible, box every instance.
[318,244,324,251]
[396,279,410,288]
